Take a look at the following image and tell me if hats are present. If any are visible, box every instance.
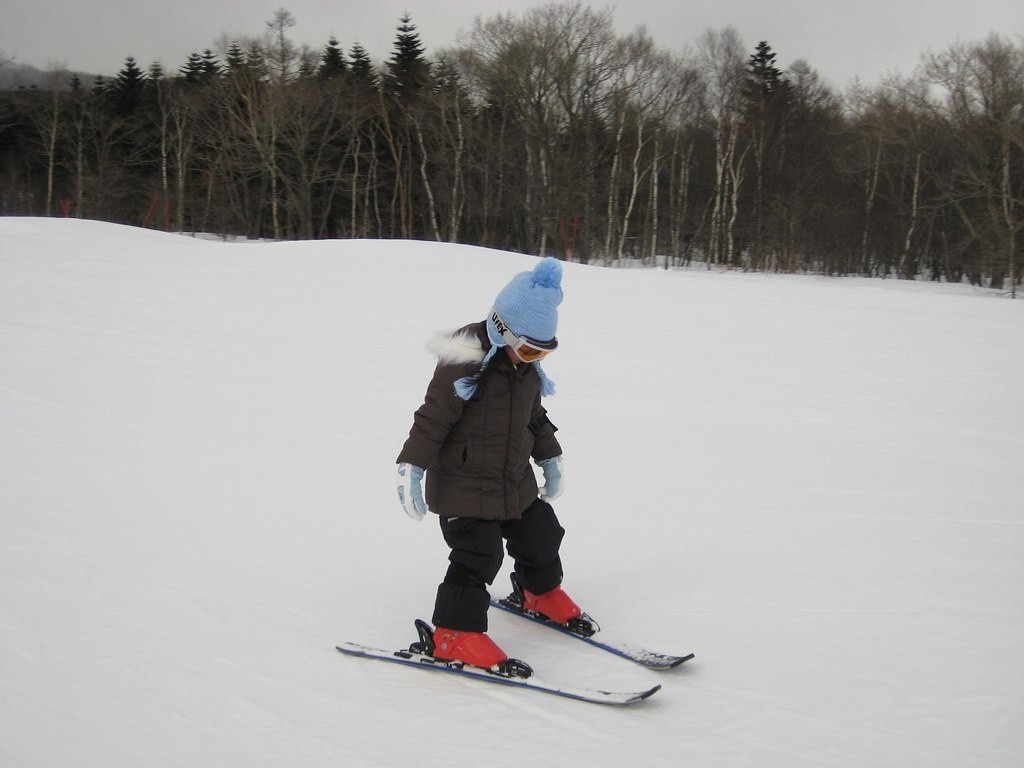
[486,257,563,347]
[538,455,566,502]
[397,462,426,521]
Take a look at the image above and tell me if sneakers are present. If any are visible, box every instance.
[433,627,508,667]
[524,585,581,623]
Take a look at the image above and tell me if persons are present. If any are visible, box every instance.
[396,258,601,680]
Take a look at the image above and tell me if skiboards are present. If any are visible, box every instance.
[459,570,695,671]
[334,640,660,707]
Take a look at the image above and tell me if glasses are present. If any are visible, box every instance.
[489,306,559,363]
